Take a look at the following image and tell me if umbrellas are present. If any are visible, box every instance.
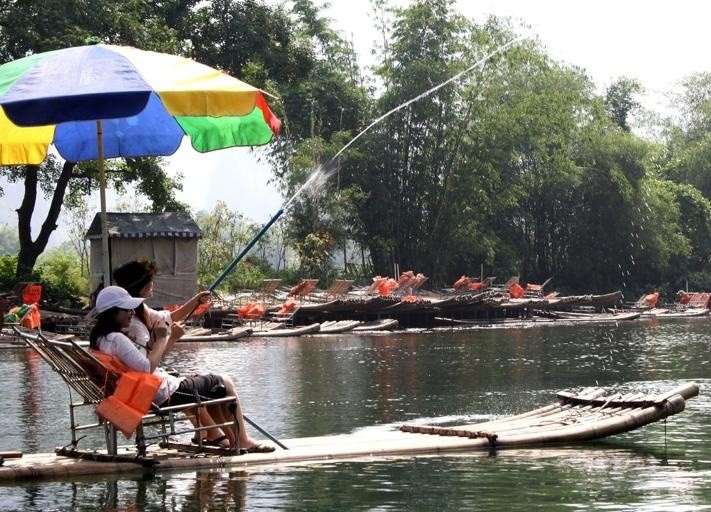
[0,44,282,453]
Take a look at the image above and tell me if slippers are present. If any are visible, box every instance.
[240,443,275,454]
[191,435,228,446]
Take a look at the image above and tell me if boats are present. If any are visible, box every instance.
[0,377,701,483]
[171,271,709,338]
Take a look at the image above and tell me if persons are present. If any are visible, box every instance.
[89,285,277,453]
[114,259,232,450]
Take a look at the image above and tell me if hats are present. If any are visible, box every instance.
[96,286,146,314]
